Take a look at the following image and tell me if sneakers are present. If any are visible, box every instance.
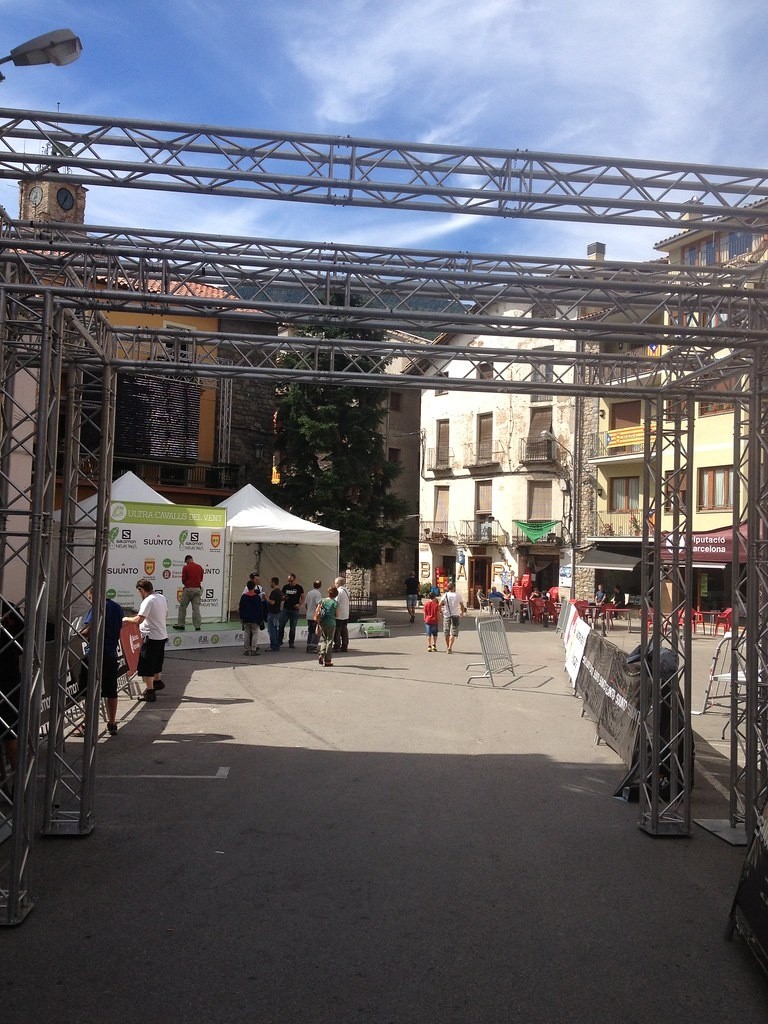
[68,724,87,737]
[318,655,324,665]
[324,660,333,666]
[152,680,165,691]
[137,689,156,702]
[105,722,118,735]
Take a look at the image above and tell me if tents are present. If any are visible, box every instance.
[48,470,340,623]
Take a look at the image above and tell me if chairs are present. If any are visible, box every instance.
[639,607,653,634]
[480,574,616,632]
[665,605,685,635]
[715,608,733,637]
[691,608,705,636]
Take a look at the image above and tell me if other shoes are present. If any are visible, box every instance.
[447,648,454,654]
[196,627,201,631]
[620,615,624,619]
[332,645,341,652]
[340,645,348,652]
[243,651,250,656]
[306,649,319,654]
[428,648,432,652]
[614,617,618,620]
[288,645,295,648]
[279,641,284,645]
[249,651,261,656]
[410,615,415,623]
[265,647,275,651]
[173,625,185,630]
[432,646,437,651]
[256,647,260,650]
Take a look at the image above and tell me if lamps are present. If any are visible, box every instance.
[541,430,573,464]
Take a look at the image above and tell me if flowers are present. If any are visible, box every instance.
[629,515,641,530]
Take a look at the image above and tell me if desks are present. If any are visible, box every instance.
[697,611,727,638]
[582,606,602,626]
[605,608,631,634]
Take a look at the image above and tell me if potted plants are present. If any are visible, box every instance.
[420,583,436,605]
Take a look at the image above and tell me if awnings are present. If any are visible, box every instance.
[660,515,768,563]
[575,542,654,572]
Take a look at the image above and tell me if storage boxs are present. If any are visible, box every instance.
[436,567,448,595]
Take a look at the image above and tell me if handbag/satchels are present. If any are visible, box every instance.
[318,606,329,621]
[315,624,321,637]
[313,604,321,623]
[451,617,460,626]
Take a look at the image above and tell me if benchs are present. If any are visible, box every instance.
[362,629,391,639]
[357,617,385,623]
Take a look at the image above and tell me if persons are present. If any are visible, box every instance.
[0,596,26,803]
[527,588,549,601]
[588,585,625,620]
[172,556,203,631]
[476,584,515,618]
[77,578,168,736]
[404,570,420,624]
[239,573,349,667]
[422,582,464,654]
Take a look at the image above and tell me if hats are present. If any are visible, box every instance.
[185,555,193,562]
[250,573,260,578]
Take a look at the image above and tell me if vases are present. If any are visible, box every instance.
[634,530,640,536]
